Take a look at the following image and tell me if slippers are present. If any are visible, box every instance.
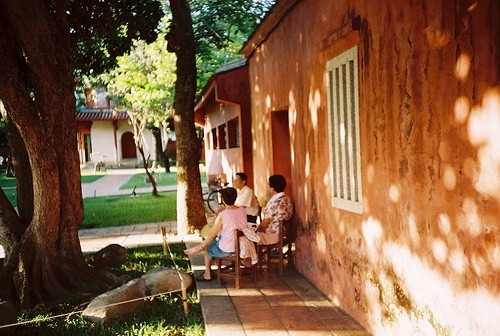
[196,270,216,282]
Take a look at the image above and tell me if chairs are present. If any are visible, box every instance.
[255,220,294,275]
[215,225,259,288]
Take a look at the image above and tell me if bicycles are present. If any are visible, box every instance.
[207,178,229,215]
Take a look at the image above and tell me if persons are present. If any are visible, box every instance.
[182,187,248,282]
[234,172,259,223]
[257,174,293,246]
